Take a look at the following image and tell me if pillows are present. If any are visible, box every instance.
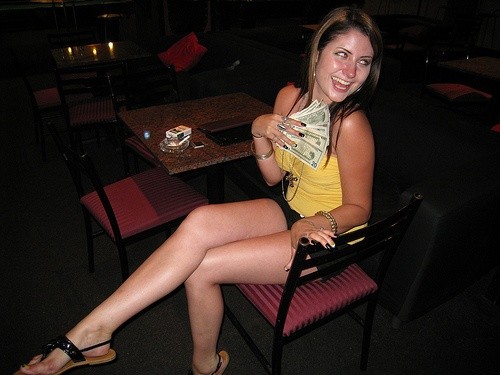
[157,31,209,75]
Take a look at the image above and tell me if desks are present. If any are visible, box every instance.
[49,39,152,145]
[116,92,273,203]
[436,56,500,81]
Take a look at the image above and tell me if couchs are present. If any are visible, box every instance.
[148,29,500,322]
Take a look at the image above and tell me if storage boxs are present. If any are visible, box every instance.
[166,125,192,140]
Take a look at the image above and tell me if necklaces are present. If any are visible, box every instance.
[281,91,309,202]
[286,153,298,187]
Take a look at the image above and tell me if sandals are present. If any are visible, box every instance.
[14,335,116,375]
[190,350,229,375]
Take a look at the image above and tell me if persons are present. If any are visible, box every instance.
[13,7,383,375]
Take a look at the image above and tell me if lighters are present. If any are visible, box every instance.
[191,140,205,149]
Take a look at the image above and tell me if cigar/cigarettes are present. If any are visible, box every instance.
[179,135,191,145]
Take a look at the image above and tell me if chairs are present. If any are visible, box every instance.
[8,1,500,375]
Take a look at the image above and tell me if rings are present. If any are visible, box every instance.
[278,124,287,131]
[308,231,315,239]
[319,227,325,233]
[281,116,289,124]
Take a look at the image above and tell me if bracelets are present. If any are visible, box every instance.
[314,210,338,233]
[251,140,274,159]
